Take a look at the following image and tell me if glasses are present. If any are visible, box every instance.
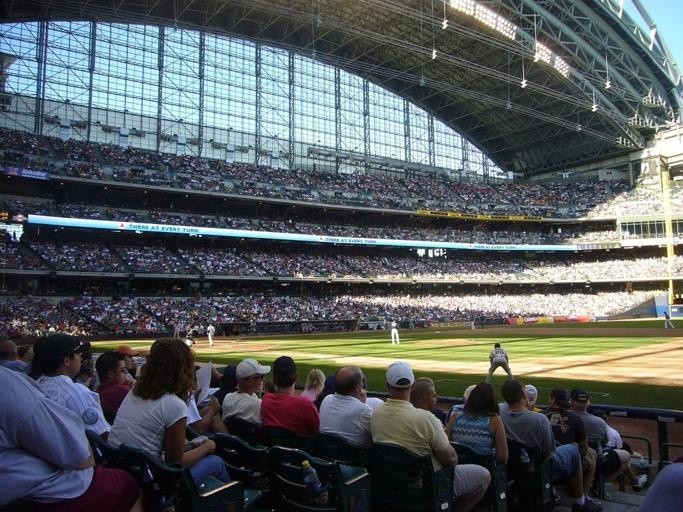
[246,375,264,381]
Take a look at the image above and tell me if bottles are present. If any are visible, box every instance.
[640,455,646,468]
[520,448,530,463]
[301,460,321,489]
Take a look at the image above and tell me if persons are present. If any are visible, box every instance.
[0,125,682,511]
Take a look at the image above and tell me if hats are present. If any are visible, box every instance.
[38,334,90,354]
[525,384,537,401]
[116,346,140,356]
[551,389,588,408]
[386,362,415,388]
[224,358,271,378]
[325,376,335,391]
[273,356,295,375]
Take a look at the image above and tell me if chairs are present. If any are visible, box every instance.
[83,415,626,512]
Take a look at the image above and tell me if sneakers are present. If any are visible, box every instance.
[554,487,616,512]
[632,474,647,492]
